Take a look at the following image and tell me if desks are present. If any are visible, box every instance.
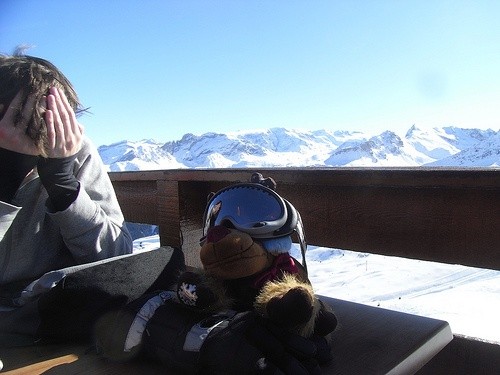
[1,263,454,374]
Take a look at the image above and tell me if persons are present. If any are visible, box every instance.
[203,200,223,237]
[0,43,134,373]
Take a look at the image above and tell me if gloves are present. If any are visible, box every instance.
[123,272,337,375]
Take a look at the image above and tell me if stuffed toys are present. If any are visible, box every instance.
[198,173,339,364]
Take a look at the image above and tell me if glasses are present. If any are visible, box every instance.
[203,182,308,281]
